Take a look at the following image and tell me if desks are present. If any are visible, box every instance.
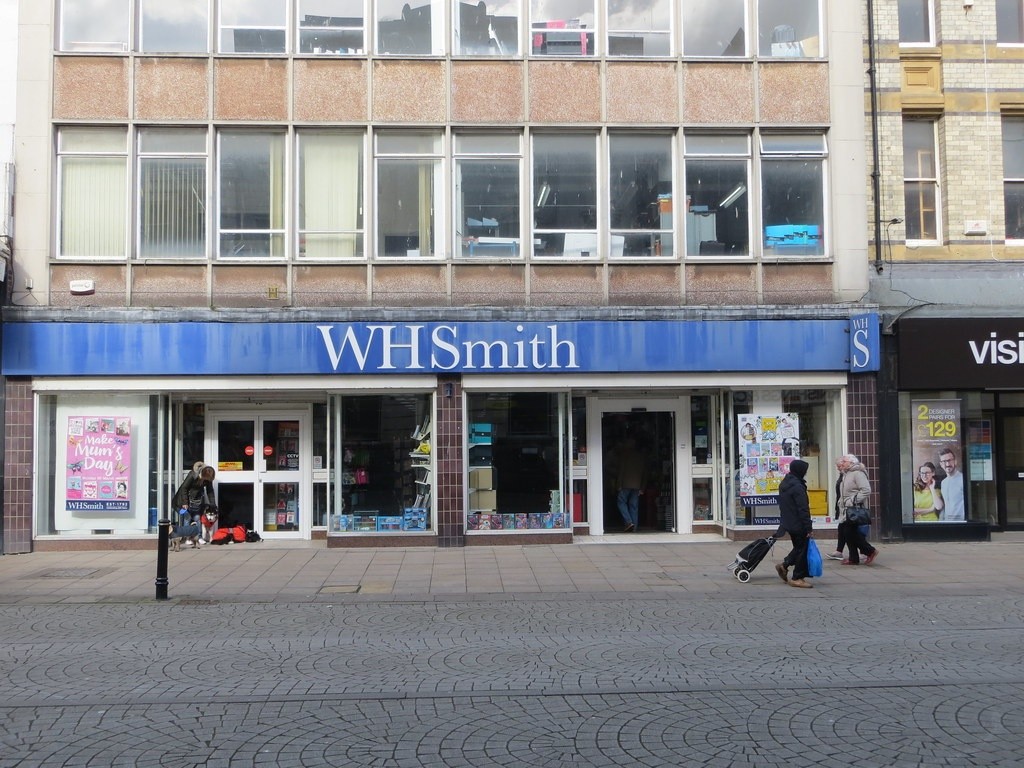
[464,225,500,237]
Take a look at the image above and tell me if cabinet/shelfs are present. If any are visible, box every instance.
[393,433,416,506]
[410,453,431,485]
[660,212,720,255]
[917,150,936,239]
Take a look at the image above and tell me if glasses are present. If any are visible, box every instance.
[919,471,932,476]
[940,458,954,464]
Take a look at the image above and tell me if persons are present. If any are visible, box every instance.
[742,423,751,436]
[617,439,649,532]
[775,460,815,587]
[743,483,751,495]
[172,462,216,545]
[782,418,794,430]
[825,454,879,565]
[914,449,964,521]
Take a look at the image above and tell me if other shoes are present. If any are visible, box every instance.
[624,522,634,532]
[631,527,637,532]
[864,550,879,565]
[840,559,859,565]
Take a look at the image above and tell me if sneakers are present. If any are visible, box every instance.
[775,563,789,582]
[185,540,193,545]
[825,552,844,561]
[790,579,812,588]
[197,538,206,545]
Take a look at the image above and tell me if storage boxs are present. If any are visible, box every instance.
[467,514,567,529]
[766,225,818,244]
[587,36,645,55]
[469,423,494,442]
[636,192,691,226]
[468,466,498,490]
[468,489,497,511]
[331,508,428,532]
[406,247,420,256]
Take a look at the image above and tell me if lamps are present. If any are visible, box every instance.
[719,185,747,210]
[537,183,550,208]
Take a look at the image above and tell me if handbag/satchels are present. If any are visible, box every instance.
[188,499,203,513]
[846,494,871,526]
[807,538,823,576]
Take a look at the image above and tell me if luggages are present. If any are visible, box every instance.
[727,536,776,582]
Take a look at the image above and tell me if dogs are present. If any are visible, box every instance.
[210,523,265,546]
[200,503,219,543]
[169,522,200,552]
